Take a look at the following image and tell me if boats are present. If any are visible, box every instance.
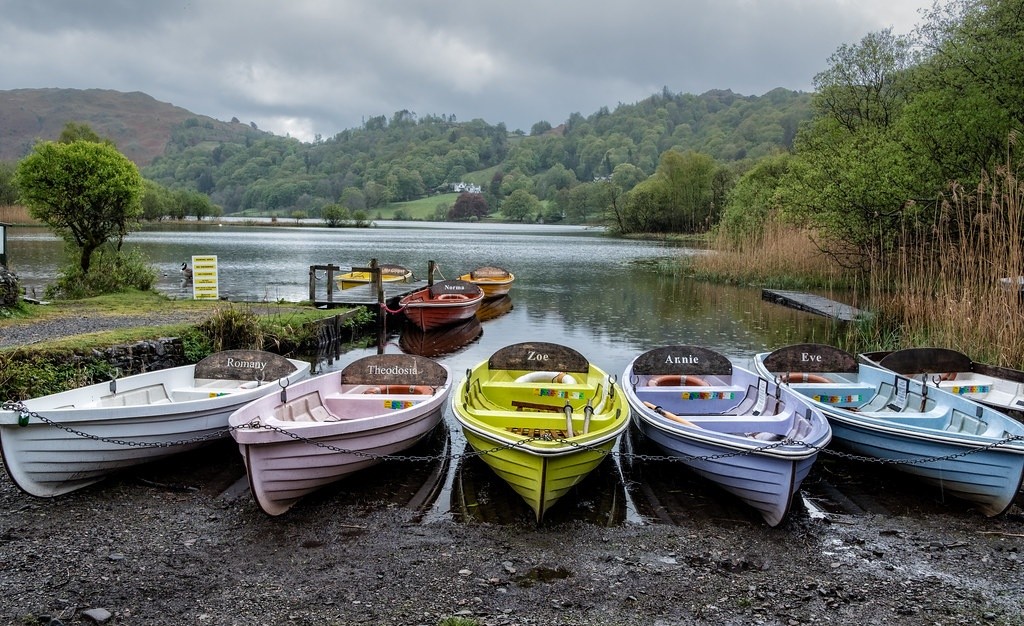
[857,346,1024,420]
[398,279,485,333]
[751,342,1024,518]
[456,265,515,299]
[0,349,313,499]
[333,262,413,291]
[621,342,835,529]
[448,342,632,527]
[226,354,455,518]
